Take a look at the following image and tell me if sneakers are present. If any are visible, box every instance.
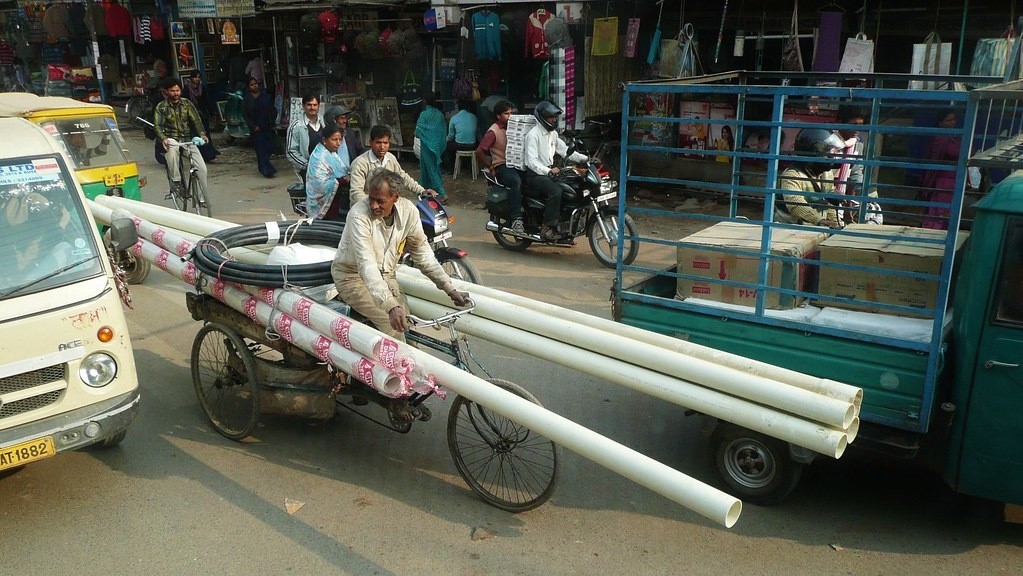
[510,218,524,232]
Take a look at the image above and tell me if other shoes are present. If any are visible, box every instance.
[193,200,207,208]
[170,183,181,193]
[440,195,449,203]
[540,224,561,240]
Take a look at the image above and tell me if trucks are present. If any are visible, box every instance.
[610,69,1023,510]
[0,116,140,480]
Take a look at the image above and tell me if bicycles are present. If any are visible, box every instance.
[164,141,211,218]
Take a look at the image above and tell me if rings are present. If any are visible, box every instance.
[396,326,398,329]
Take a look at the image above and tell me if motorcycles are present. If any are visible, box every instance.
[0,92,151,285]
[480,130,639,269]
[287,183,480,286]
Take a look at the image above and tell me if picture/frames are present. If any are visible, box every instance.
[170,17,230,92]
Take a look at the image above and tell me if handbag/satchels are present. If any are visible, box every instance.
[453,67,480,102]
[440,54,457,82]
[781,34,803,73]
[400,69,422,106]
[838,27,1023,88]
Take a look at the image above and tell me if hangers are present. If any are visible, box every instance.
[134,12,161,18]
[48,1,127,8]
[530,4,555,16]
[473,4,496,16]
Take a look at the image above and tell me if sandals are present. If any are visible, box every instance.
[390,400,431,423]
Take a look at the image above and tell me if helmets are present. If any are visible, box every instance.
[794,126,834,174]
[535,101,564,131]
[324,106,351,128]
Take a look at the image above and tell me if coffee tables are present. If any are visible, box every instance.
[452,149,479,181]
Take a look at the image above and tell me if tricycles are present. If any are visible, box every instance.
[184,291,563,512]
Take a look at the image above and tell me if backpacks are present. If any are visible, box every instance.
[300,11,424,62]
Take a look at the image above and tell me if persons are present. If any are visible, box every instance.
[715,125,734,162]
[183,69,221,164]
[306,123,350,220]
[152,78,166,123]
[774,126,847,229]
[443,100,480,175]
[180,42,190,69]
[414,92,448,204]
[242,77,278,177]
[309,105,367,163]
[482,96,518,124]
[524,100,601,240]
[331,167,470,422]
[1,183,84,288]
[64,119,111,167]
[153,75,211,208]
[286,95,329,184]
[350,125,438,211]
[824,102,864,224]
[918,112,976,232]
[690,117,705,140]
[475,101,524,232]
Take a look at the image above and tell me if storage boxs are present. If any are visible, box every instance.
[675,220,830,312]
[505,115,536,172]
[814,220,973,318]
